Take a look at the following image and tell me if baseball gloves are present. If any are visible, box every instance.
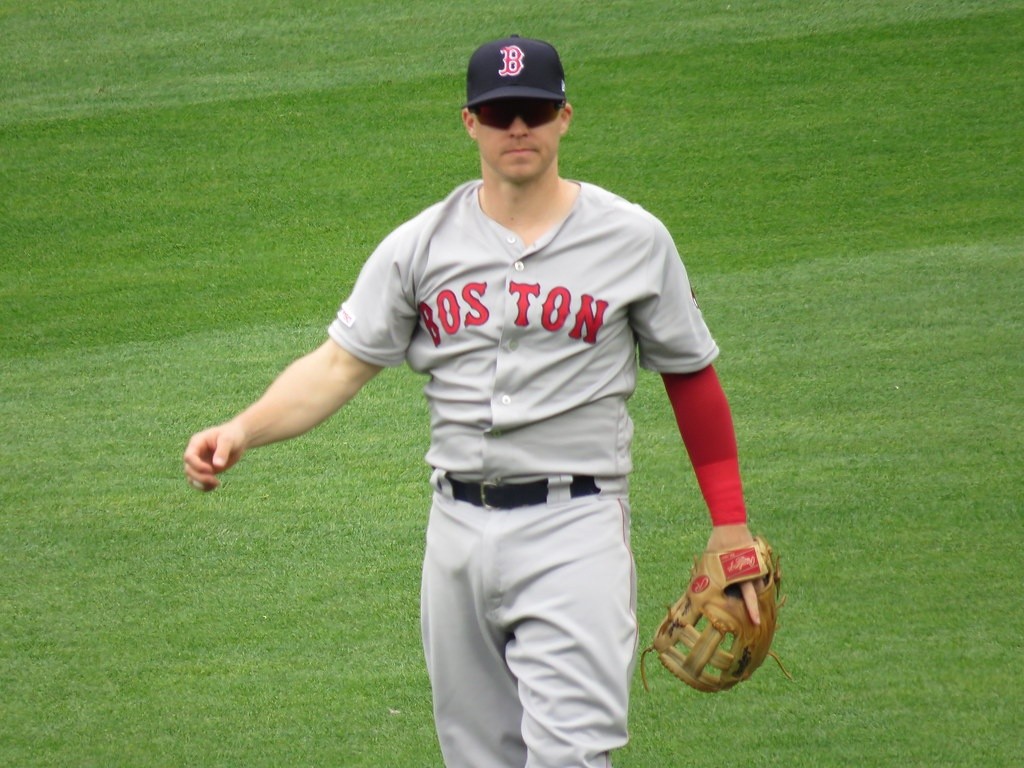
[651,534,778,693]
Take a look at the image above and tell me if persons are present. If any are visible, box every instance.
[185,36,775,768]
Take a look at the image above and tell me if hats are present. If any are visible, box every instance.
[460,35,566,107]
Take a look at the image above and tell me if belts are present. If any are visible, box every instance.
[431,467,601,510]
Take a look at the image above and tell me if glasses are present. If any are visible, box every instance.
[468,97,565,130]
